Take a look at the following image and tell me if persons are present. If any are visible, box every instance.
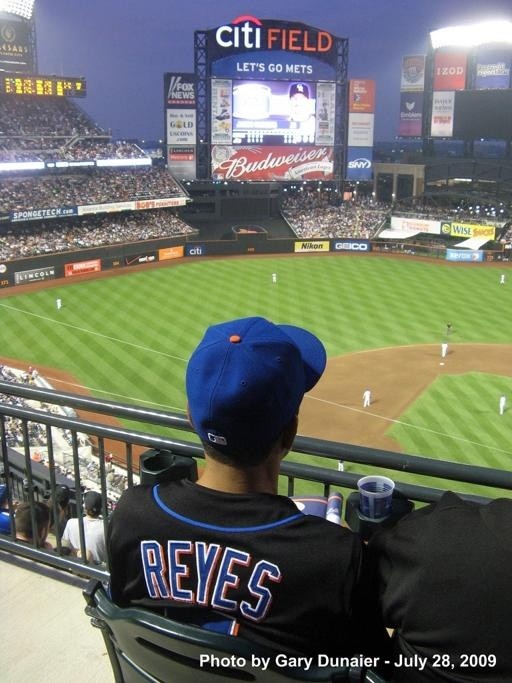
[366,487,511,683]
[55,296,63,311]
[362,388,371,407]
[337,458,345,472]
[102,314,396,681]
[0,363,139,581]
[440,340,448,359]
[499,393,507,414]
[271,271,278,286]
[277,181,512,262]
[1,89,200,263]
[497,272,506,289]
[285,83,312,121]
[446,321,452,333]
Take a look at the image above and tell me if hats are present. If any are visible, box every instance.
[0,485,6,499]
[55,484,70,501]
[187,317,327,447]
[82,491,102,512]
[288,81,310,100]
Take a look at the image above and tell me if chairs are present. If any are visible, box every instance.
[83,579,367,683]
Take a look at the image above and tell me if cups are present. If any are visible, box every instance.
[357,476,395,518]
